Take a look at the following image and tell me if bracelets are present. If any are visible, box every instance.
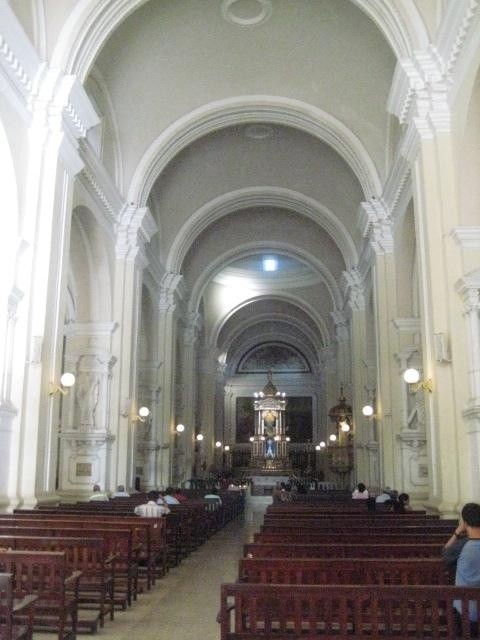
[453,532,460,539]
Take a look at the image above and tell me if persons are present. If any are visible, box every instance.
[111,484,130,499]
[375,487,391,502]
[391,493,412,511]
[278,479,308,503]
[439,503,480,640]
[203,487,222,513]
[134,490,171,517]
[352,483,368,499]
[384,490,398,504]
[90,485,109,501]
[173,488,189,500]
[157,486,181,505]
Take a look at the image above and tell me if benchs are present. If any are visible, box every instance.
[221,488,479,640]
[1,484,220,640]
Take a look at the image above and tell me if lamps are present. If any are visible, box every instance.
[170,367,434,452]
[46,372,76,398]
[130,406,151,424]
